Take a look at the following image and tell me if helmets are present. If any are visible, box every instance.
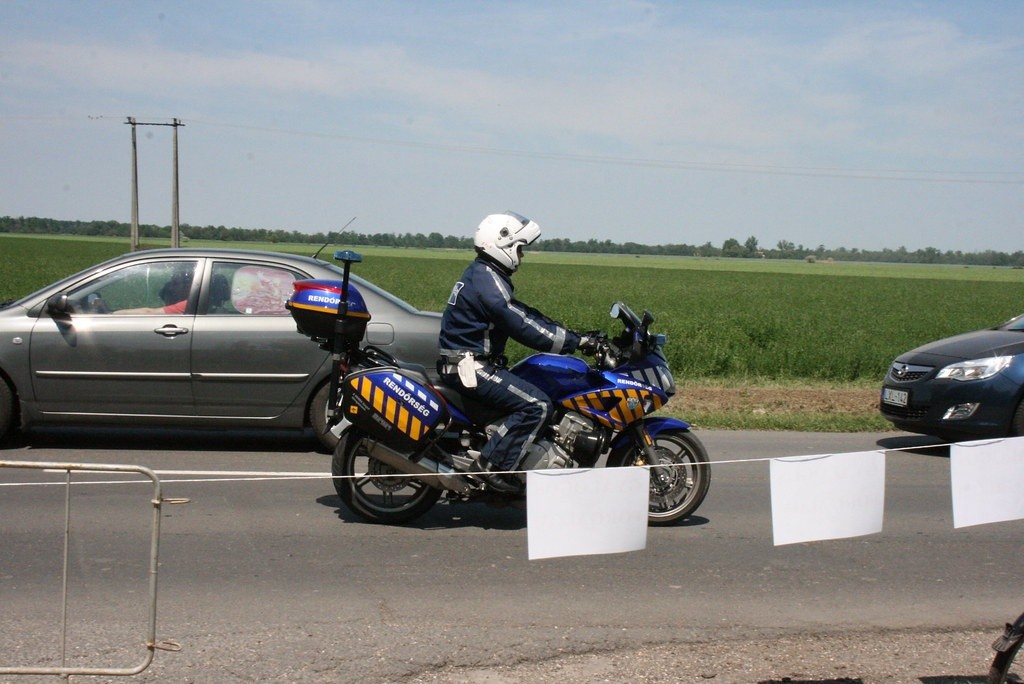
[473,210,541,276]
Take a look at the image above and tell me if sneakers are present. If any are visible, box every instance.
[468,455,522,494]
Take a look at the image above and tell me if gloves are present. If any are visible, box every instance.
[576,336,609,357]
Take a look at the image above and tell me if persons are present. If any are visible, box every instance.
[106,270,193,315]
[436,211,597,493]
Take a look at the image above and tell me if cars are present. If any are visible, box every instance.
[877,310,1024,449]
[0,247,441,460]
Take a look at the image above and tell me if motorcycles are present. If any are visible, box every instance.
[285,251,712,527]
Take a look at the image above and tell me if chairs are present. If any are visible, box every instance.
[210,271,231,314]
